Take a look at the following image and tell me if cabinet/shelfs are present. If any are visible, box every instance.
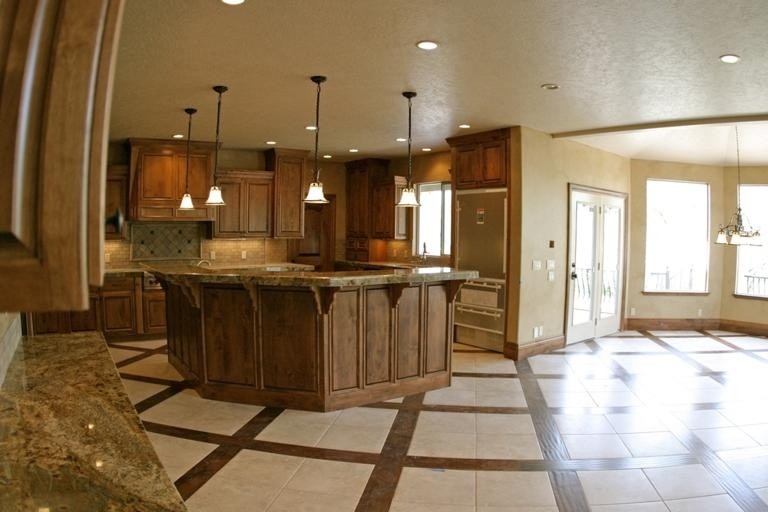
[445,128,511,190]
[104,164,127,240]
[144,287,167,338]
[0,1,127,309]
[369,175,409,240]
[264,148,311,240]
[71,272,145,339]
[31,310,72,336]
[345,157,389,261]
[127,136,224,223]
[210,168,275,240]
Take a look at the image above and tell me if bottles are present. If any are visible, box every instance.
[422,242,428,266]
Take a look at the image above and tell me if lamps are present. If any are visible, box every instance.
[715,126,763,245]
[204,85,228,206]
[178,107,198,211]
[395,91,421,208]
[299,75,331,205]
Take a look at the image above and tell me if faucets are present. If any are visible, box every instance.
[423,242,428,259]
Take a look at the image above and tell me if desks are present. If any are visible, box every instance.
[0,331,188,512]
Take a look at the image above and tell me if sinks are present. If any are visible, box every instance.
[408,261,432,264]
